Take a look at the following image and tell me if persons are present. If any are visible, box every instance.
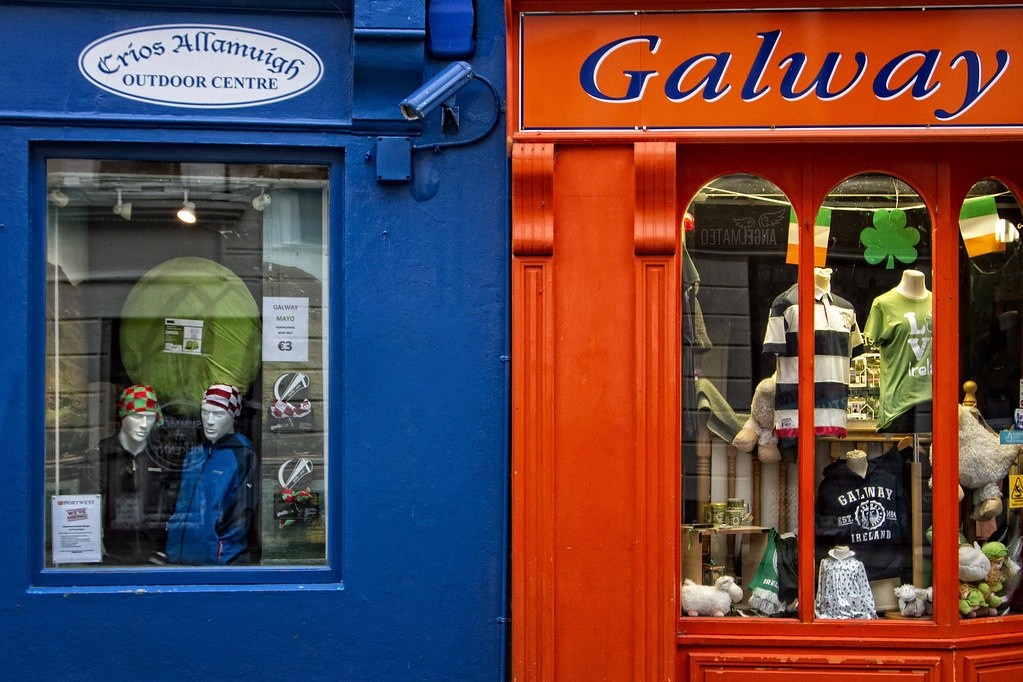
[165,383,258,565]
[863,270,932,433]
[815,545,877,619]
[79,383,173,567]
[814,450,910,612]
[762,268,866,437]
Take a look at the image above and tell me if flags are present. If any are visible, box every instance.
[785,206,832,267]
[958,195,1006,258]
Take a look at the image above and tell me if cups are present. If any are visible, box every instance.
[704,498,752,527]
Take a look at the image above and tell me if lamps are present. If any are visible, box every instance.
[48,181,69,207]
[252,184,271,211]
[176,188,197,224]
[113,187,132,220]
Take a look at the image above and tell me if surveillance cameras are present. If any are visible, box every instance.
[398,61,474,122]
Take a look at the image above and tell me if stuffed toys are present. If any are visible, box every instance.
[926,524,1021,619]
[928,404,1019,522]
[680,575,743,616]
[893,582,927,616]
[732,370,781,462]
